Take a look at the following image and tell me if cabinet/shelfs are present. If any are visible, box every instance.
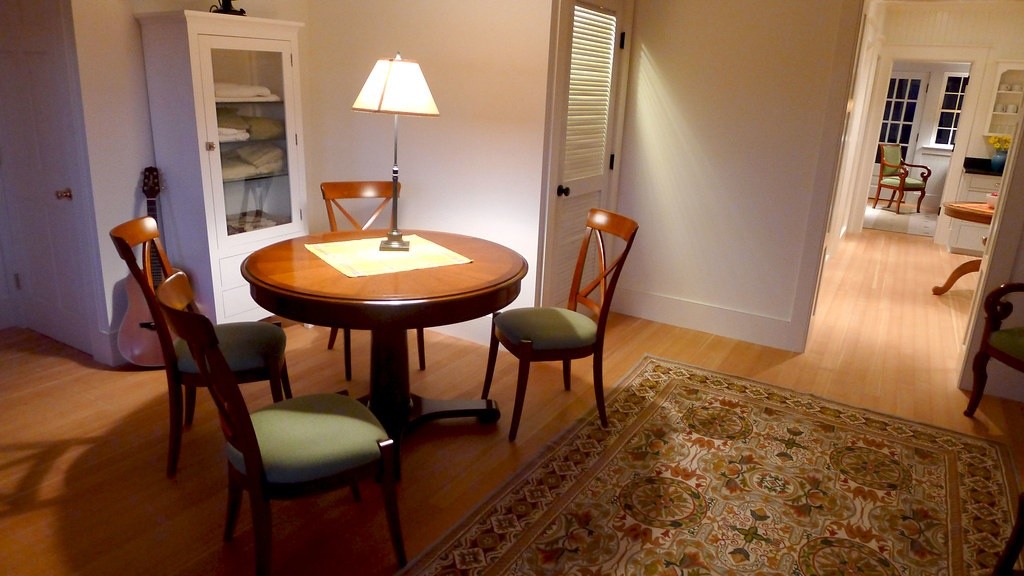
[984,59,1024,137]
[132,9,309,324]
[949,171,1002,257]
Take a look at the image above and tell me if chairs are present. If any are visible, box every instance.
[157,271,408,576]
[964,282,1024,417]
[872,143,931,215]
[481,209,639,443]
[320,181,425,381]
[108,215,292,479]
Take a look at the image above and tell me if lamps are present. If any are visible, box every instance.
[351,52,441,250]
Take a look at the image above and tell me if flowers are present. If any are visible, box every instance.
[988,135,1012,151]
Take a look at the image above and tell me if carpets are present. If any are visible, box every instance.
[396,353,1024,576]
[862,201,938,237]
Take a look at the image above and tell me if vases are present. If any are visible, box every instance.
[990,148,1007,174]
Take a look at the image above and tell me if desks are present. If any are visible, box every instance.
[932,202,996,295]
[240,230,528,485]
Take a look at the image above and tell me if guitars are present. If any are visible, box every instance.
[116,165,205,368]
